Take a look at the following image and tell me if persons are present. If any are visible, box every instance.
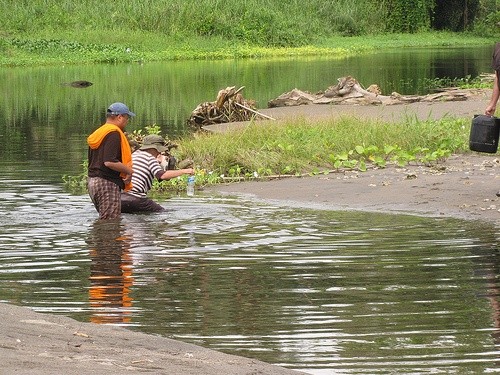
[485,38,500,199]
[119,134,195,214]
[86,102,135,220]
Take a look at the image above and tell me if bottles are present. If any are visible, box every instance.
[187,174,195,197]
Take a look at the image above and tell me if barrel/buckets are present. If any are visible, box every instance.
[469,114,500,154]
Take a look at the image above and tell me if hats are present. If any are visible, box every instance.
[106,102,136,117]
[139,134,169,152]
